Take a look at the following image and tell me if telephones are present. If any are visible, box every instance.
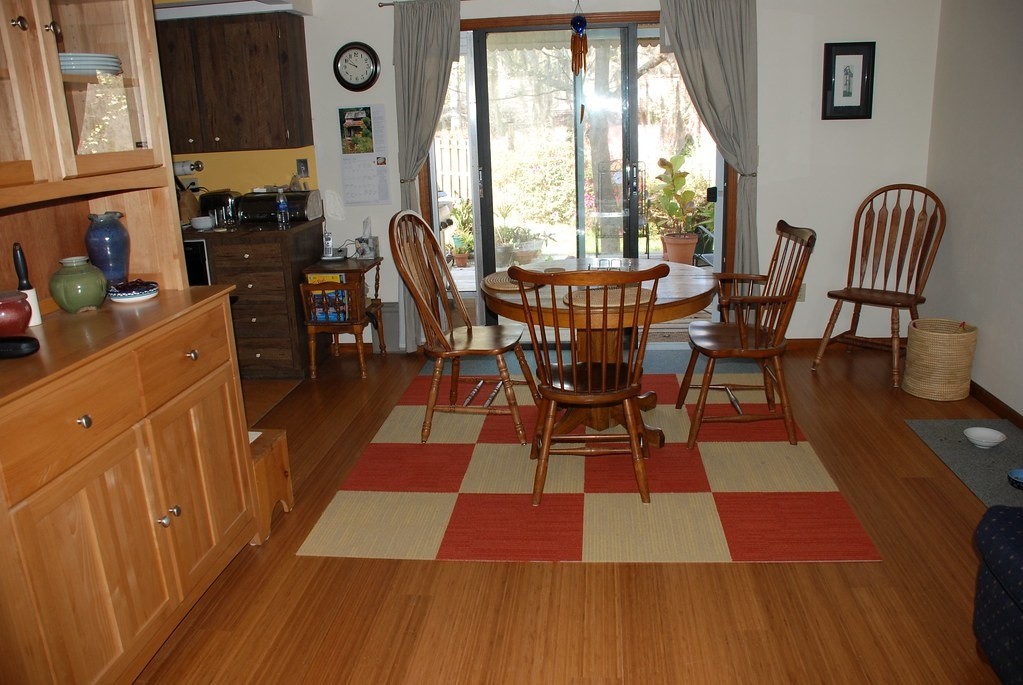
[320,231,347,262]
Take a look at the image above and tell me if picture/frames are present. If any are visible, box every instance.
[821,41,877,118]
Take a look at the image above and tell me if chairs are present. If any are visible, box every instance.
[389,209,541,445]
[676,220,816,449]
[810,183,946,388]
[593,160,650,256]
[299,282,387,379]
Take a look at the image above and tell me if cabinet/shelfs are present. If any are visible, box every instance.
[155,12,313,155]
[0,0,259,685]
[182,216,326,379]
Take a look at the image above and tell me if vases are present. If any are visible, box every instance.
[85,210,130,289]
[51,256,107,313]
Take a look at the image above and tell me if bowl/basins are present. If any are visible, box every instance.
[1007,469,1023,490]
[190,216,213,230]
[963,427,1007,449]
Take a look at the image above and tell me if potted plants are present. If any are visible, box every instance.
[447,190,555,268]
[650,135,715,265]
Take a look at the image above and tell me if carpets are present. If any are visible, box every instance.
[904,418,1023,510]
[295,337,886,566]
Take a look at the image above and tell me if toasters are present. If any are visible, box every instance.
[198,189,242,231]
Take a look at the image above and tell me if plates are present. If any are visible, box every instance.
[58,52,123,76]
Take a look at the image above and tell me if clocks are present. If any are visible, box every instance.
[333,41,381,93]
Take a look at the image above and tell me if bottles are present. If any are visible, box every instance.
[275,188,289,225]
[48,256,107,314]
[85,211,130,290]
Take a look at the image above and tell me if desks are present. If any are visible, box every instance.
[302,256,385,314]
[480,255,719,506]
